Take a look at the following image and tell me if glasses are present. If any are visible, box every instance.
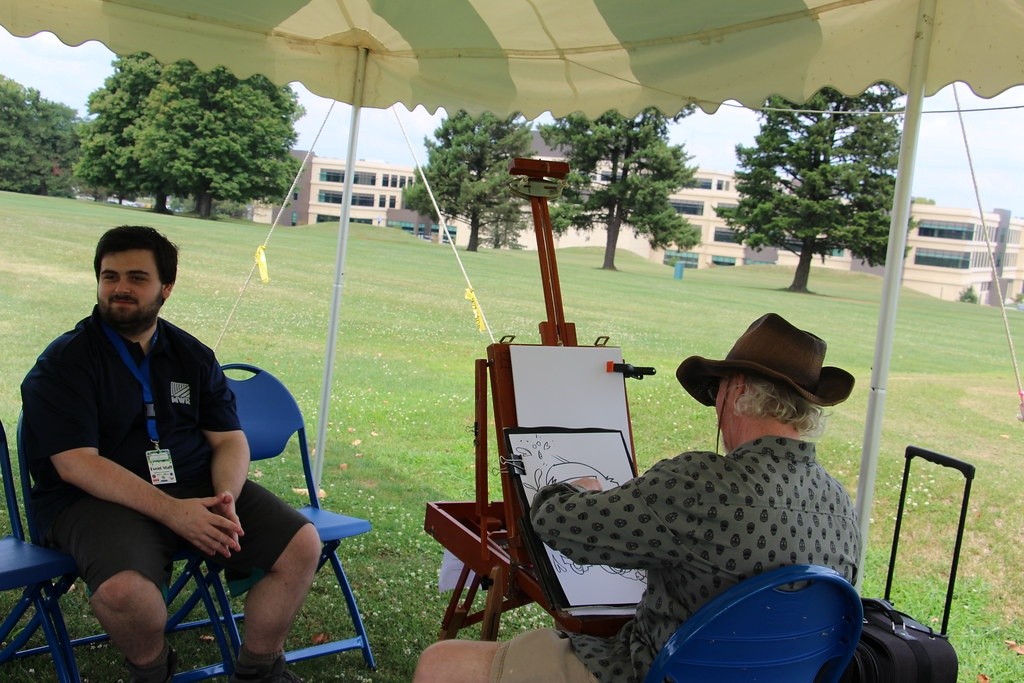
[708,379,727,402]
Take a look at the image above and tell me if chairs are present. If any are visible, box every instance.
[0,363,378,683]
[644,564,864,683]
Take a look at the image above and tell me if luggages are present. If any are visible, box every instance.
[828,445,976,683]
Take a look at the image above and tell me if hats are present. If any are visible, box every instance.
[676,312,857,406]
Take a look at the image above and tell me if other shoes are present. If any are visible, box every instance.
[235,644,300,683]
[124,643,178,683]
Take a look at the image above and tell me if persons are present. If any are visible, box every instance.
[19,226,323,683]
[409,313,864,683]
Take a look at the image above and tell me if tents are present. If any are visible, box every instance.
[0,1,1023,592]
